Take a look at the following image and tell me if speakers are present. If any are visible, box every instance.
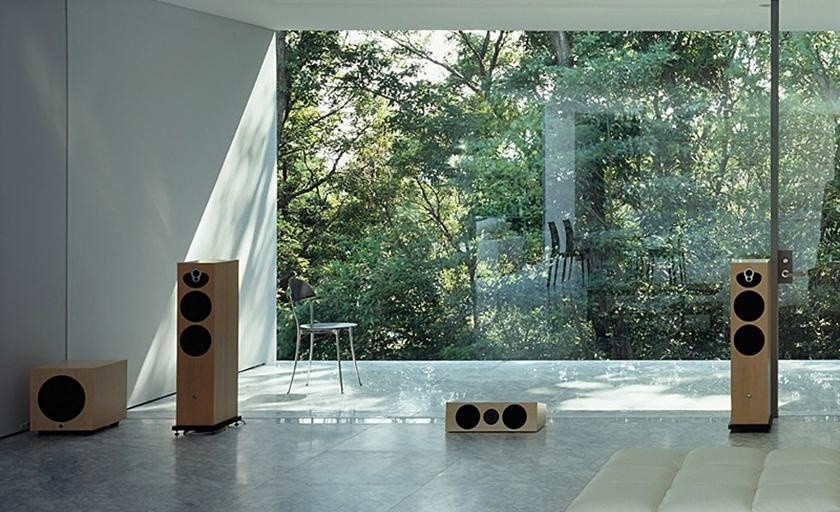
[777,248,793,283]
[446,402,547,432]
[731,258,772,425]
[30,359,127,431]
[177,257,238,426]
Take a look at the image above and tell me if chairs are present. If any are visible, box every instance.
[645,222,689,286]
[546,217,590,287]
[286,276,363,396]
